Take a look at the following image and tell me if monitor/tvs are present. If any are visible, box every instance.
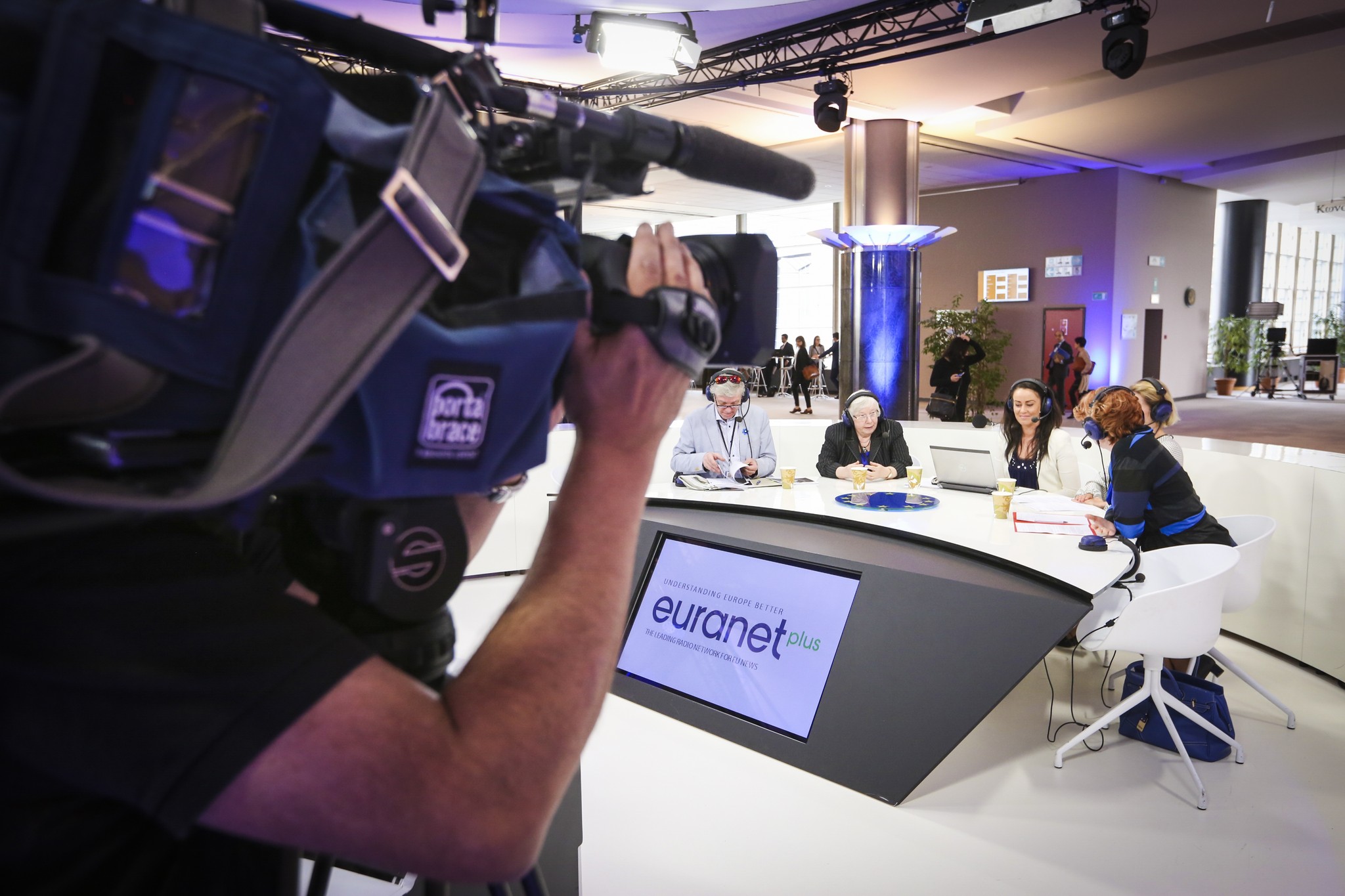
[607,528,861,744]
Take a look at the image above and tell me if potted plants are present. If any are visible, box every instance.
[1206,311,1256,396]
[1310,366,1320,388]
[1311,300,1345,385]
[1251,319,1288,395]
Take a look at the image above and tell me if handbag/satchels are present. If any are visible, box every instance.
[926,392,957,420]
[1072,357,1096,375]
[1118,661,1235,762]
[802,364,820,380]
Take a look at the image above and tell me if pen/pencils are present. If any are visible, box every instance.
[1035,520,1068,523]
[757,480,761,485]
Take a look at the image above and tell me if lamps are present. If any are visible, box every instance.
[572,13,703,75]
[1100,5,1150,80]
[813,80,848,132]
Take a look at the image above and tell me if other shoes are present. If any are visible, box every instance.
[1067,414,1073,419]
[835,396,838,399]
[1057,635,1077,648]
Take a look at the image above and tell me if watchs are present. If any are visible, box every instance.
[886,468,893,479]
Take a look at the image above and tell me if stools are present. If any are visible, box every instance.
[750,366,768,396]
[775,365,793,398]
[807,365,829,397]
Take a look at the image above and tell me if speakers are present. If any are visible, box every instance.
[1267,327,1287,345]
[1306,338,1337,360]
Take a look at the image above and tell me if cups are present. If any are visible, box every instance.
[905,466,924,489]
[997,478,1017,494]
[851,467,868,490]
[991,491,1013,519]
[779,466,796,489]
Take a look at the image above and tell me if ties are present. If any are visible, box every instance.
[1049,344,1059,368]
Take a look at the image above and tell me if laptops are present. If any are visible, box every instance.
[929,445,999,495]
[773,349,783,358]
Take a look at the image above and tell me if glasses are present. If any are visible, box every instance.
[853,409,879,422]
[713,393,742,409]
[713,375,742,384]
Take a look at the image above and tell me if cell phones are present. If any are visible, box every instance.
[956,372,965,377]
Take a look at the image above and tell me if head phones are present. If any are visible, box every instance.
[1082,386,1136,439]
[706,369,749,402]
[1007,379,1052,414]
[1137,377,1172,423]
[841,392,885,427]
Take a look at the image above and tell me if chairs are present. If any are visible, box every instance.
[1052,543,1247,810]
[1106,516,1298,731]
[907,438,943,488]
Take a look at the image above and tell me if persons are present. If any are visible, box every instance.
[813,332,839,399]
[816,389,913,481]
[929,334,987,422]
[0,0,719,896]
[789,336,813,414]
[1067,337,1093,420]
[989,378,1081,497]
[671,368,776,479]
[1047,330,1074,414]
[780,334,794,394]
[809,336,827,393]
[1059,385,1237,680]
[1072,377,1184,510]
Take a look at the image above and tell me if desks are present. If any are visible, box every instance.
[810,356,831,400]
[772,356,792,398]
[545,473,1138,805]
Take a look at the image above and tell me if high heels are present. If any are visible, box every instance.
[790,408,801,413]
[801,409,812,414]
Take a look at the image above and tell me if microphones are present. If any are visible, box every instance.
[490,86,814,202]
[883,415,890,438]
[1032,405,1054,422]
[737,396,750,422]
[1112,573,1145,584]
[1081,431,1092,449]
